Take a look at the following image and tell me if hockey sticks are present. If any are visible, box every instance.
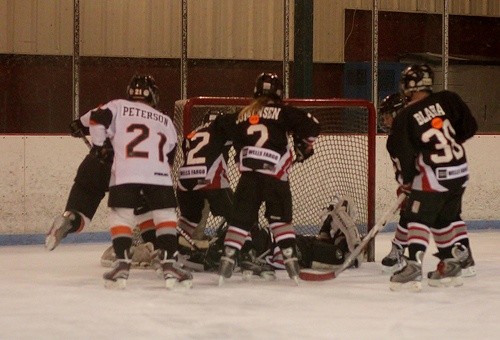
[298,194,407,282]
[79,130,93,151]
[177,226,220,268]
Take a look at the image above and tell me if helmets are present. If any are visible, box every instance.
[253,72,284,102]
[126,73,160,108]
[399,63,434,94]
[204,110,221,125]
[378,93,406,113]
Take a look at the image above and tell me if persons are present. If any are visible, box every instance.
[378,91,478,271]
[73,74,195,288]
[43,103,172,264]
[102,71,365,284]
[389,64,468,284]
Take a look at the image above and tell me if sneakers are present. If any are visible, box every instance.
[456,242,477,277]
[158,250,193,289]
[426,246,465,288]
[45,210,81,251]
[143,242,163,270]
[381,239,409,274]
[388,250,425,291]
[282,246,302,285]
[237,240,262,274]
[102,246,135,289]
[217,245,239,288]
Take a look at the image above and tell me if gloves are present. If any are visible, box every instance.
[69,119,85,138]
[397,183,411,208]
[89,144,108,160]
[294,140,314,163]
